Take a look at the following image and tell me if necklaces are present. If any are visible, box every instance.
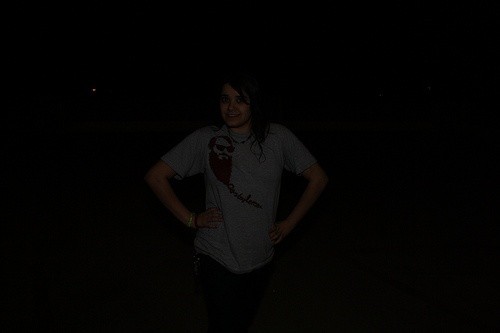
[227,128,252,144]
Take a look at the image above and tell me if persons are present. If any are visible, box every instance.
[146,73,328,333]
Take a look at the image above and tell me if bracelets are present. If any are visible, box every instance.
[187,213,199,229]
